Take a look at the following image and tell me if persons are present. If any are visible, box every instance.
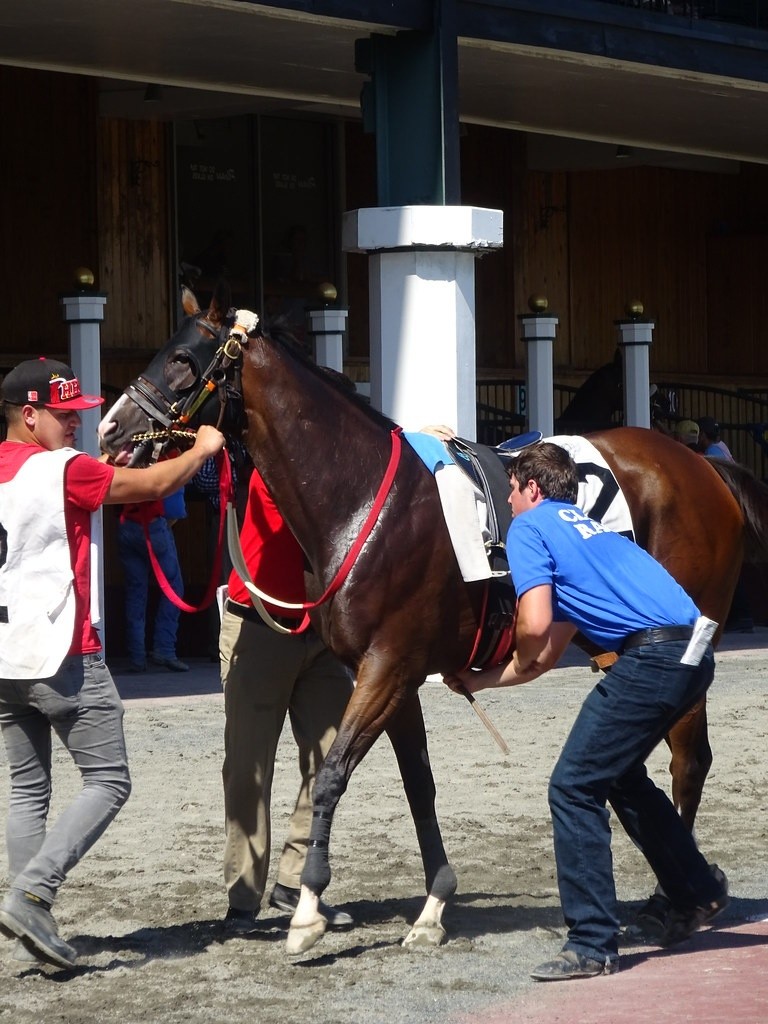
[120,443,222,671]
[442,444,734,980]
[218,466,355,931]
[655,417,756,633]
[0,356,228,967]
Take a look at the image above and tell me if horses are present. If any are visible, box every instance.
[94,284,767,958]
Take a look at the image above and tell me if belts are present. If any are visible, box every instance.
[614,625,694,658]
[227,600,316,633]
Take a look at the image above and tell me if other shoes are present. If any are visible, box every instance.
[209,648,221,664]
[150,654,190,672]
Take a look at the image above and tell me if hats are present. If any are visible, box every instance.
[1,358,105,410]
[675,420,699,444]
[699,417,721,442]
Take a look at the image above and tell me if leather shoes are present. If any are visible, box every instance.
[12,937,43,961]
[0,887,77,972]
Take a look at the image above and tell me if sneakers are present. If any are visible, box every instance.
[660,863,729,948]
[224,904,262,932]
[269,882,353,924]
[529,949,620,980]
